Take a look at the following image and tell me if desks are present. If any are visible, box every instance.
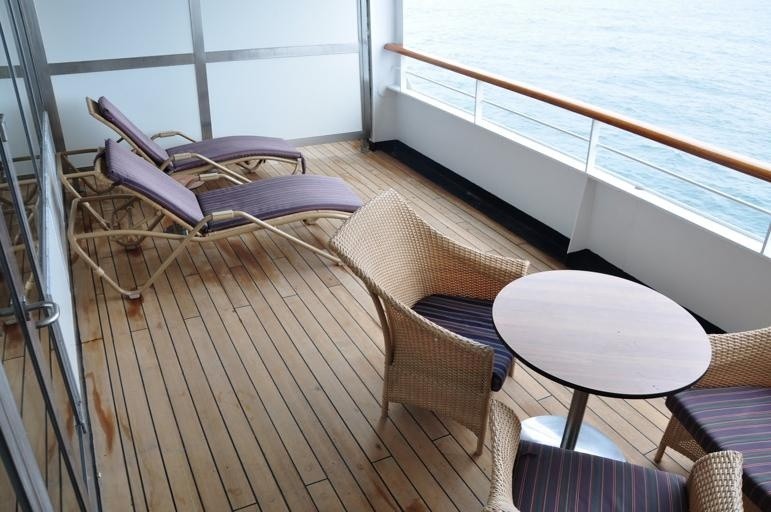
[492,269,712,462]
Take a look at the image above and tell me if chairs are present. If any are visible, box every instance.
[66,138,363,299]
[55,97,306,249]
[652,324,771,512]
[327,188,529,456]
[484,398,743,512]
